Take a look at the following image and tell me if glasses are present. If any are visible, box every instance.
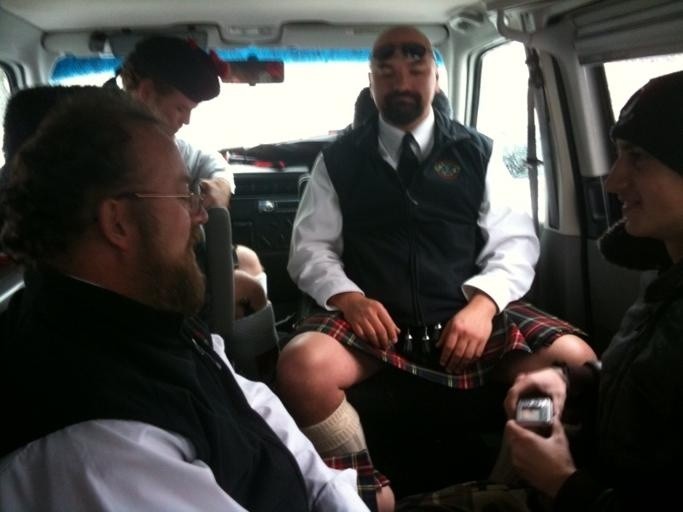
[120,182,204,216]
[372,42,433,63]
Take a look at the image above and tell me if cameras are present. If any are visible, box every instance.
[515,395,554,436]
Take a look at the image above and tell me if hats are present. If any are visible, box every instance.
[134,37,220,103]
[609,70,682,179]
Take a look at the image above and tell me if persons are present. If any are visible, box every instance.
[0,85,376,511]
[98,32,283,383]
[0,84,98,311]
[493,66,683,510]
[270,21,607,511]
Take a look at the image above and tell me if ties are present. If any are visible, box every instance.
[397,133,421,178]
